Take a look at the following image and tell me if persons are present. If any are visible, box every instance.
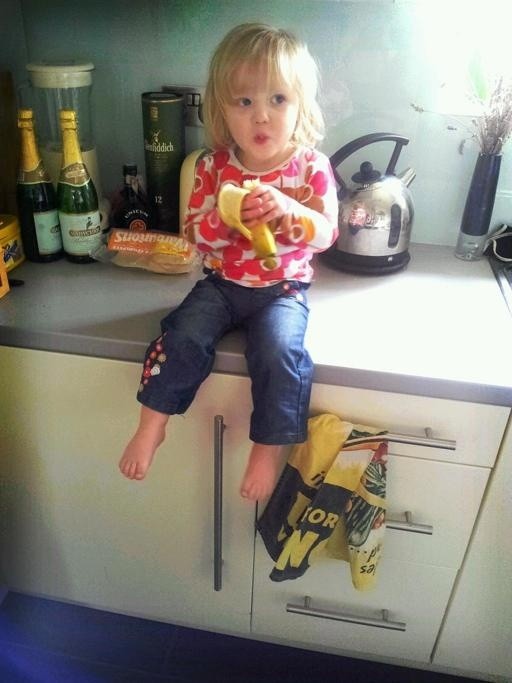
[119,22,338,501]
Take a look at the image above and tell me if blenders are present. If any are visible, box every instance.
[15,60,112,231]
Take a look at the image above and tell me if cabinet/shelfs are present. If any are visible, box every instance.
[0,226,512,683]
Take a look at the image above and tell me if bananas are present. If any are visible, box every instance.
[217,178,277,259]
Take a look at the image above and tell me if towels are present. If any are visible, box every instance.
[258,413,388,591]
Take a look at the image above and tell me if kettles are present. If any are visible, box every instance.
[316,132,416,274]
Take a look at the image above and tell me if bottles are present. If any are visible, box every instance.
[109,164,152,232]
[56,112,100,263]
[14,110,63,264]
